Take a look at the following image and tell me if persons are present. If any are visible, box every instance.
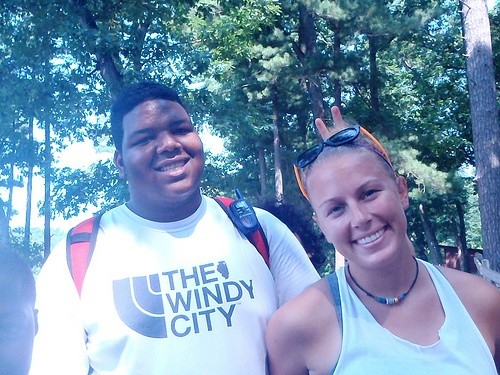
[28,81,322,375]
[0,246,39,375]
[264,124,500,375]
[441,246,495,285]
[256,105,346,270]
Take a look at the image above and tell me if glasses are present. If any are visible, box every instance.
[293,124,398,203]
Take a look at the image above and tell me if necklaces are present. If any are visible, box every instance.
[347,255,419,305]
